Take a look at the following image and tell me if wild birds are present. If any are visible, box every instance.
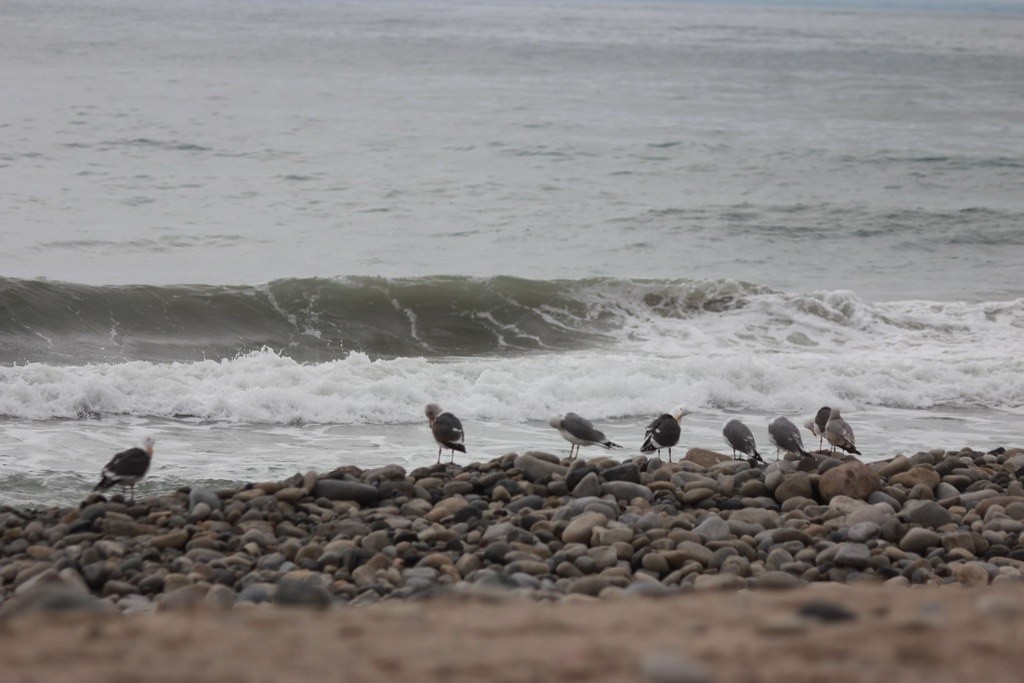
[639,405,693,465]
[90,434,160,494]
[425,402,466,464]
[767,416,816,462]
[548,410,623,461]
[721,417,765,463]
[803,405,863,460]
